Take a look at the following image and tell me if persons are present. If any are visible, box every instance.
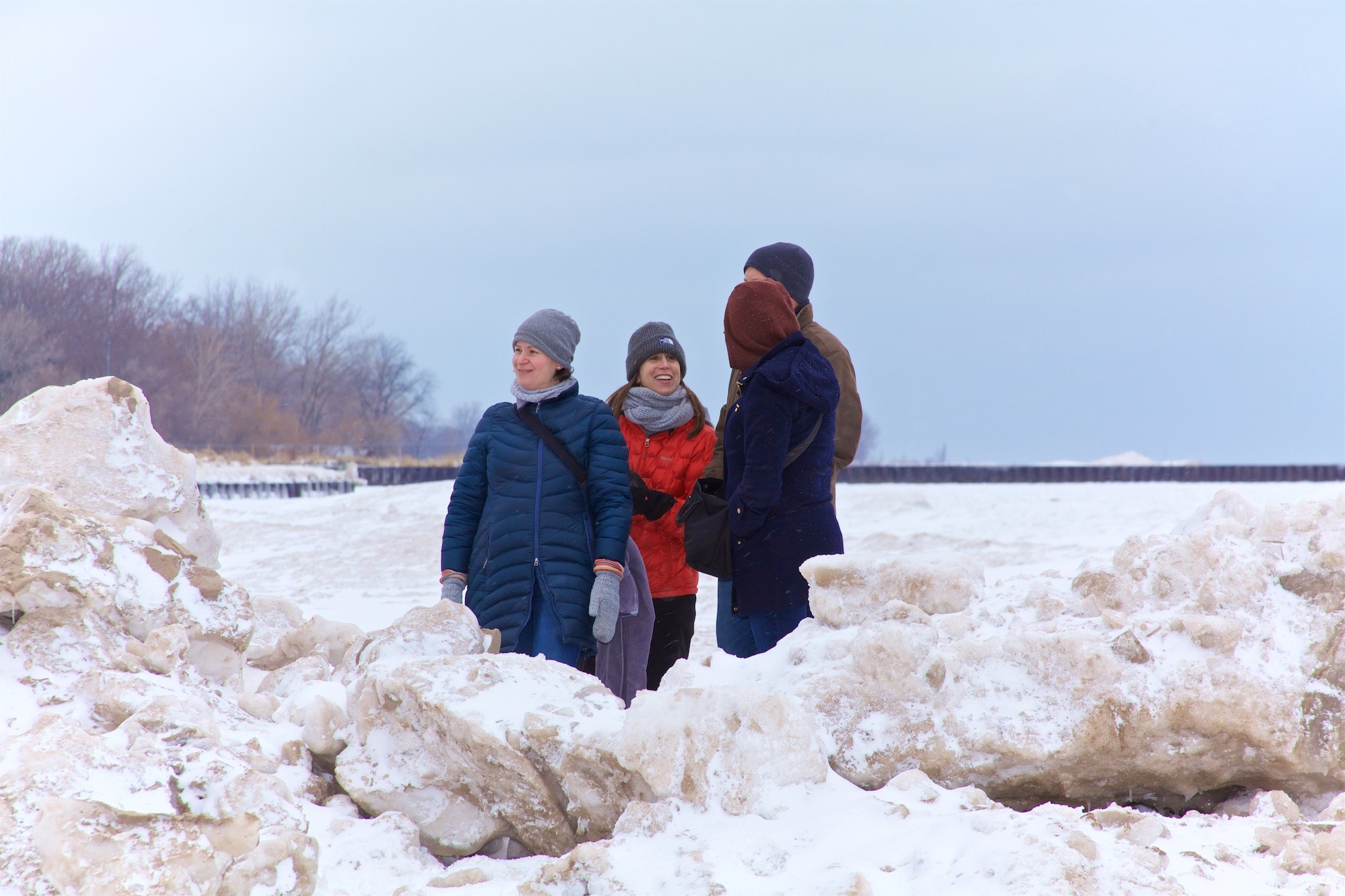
[722,279,844,652]
[602,321,717,688]
[440,308,631,671]
[702,242,862,657]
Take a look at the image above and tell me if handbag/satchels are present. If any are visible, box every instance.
[675,477,734,581]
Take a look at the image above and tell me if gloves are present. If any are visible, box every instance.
[588,570,622,644]
[628,470,676,522]
[440,578,465,604]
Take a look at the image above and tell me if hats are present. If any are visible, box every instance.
[625,321,686,381]
[744,242,814,304]
[513,308,580,371]
